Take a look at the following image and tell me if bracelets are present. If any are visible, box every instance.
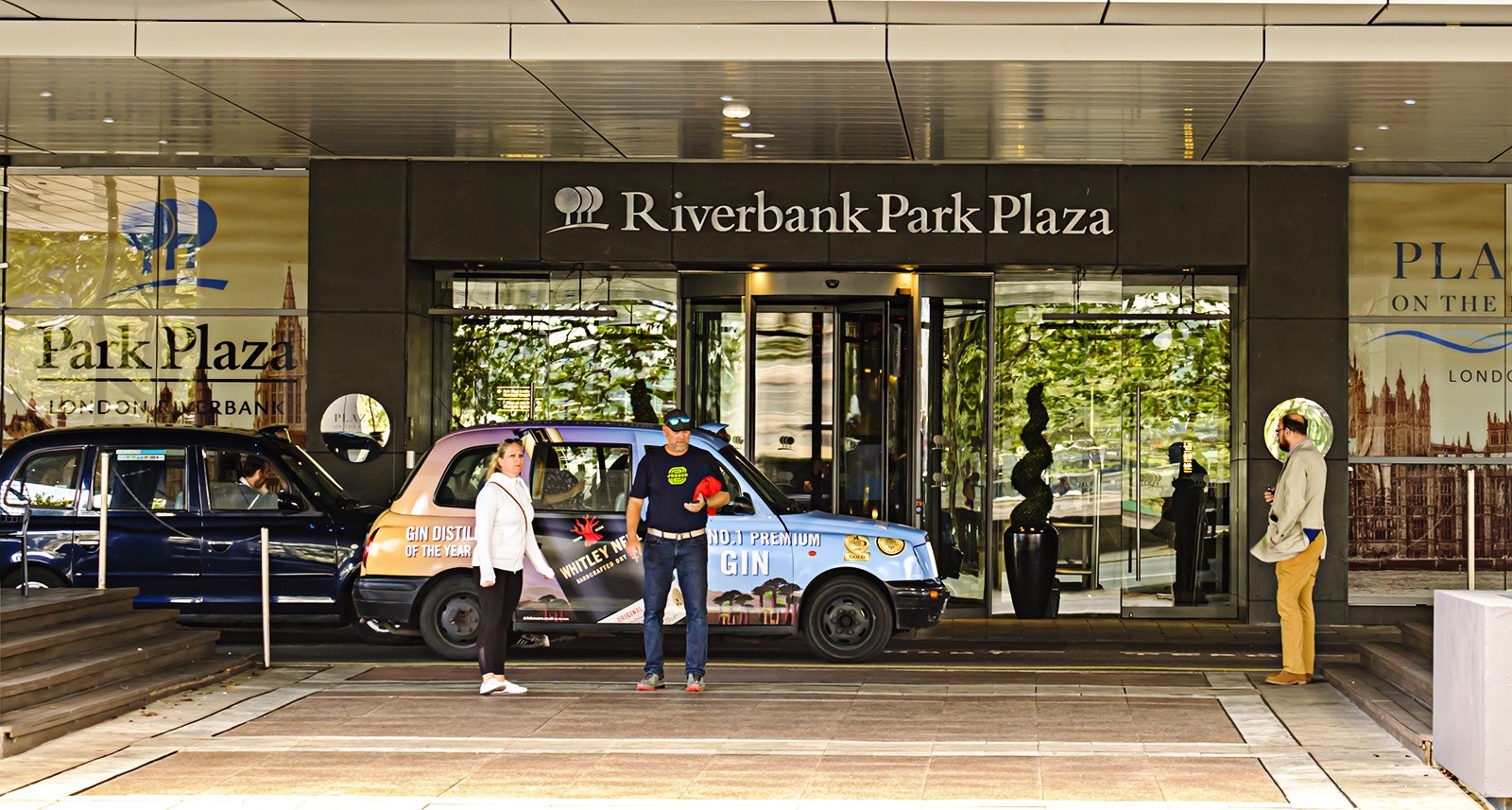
[704,498,708,508]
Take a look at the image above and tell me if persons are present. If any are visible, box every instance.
[224,454,280,510]
[628,409,730,692]
[1162,442,1211,607]
[1263,413,1328,686]
[472,437,555,696]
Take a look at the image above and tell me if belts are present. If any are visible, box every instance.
[647,528,706,540]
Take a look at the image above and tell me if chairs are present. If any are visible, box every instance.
[211,477,265,514]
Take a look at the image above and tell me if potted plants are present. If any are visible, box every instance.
[1003,379,1064,615]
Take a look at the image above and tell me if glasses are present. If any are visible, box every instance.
[499,438,523,457]
[1275,428,1284,436]
[666,416,691,425]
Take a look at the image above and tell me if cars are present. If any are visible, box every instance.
[351,425,949,664]
[0,424,426,646]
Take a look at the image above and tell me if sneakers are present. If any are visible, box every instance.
[636,671,665,691]
[490,680,528,695]
[685,673,704,692]
[480,679,506,694]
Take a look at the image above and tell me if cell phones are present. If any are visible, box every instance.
[690,500,700,503]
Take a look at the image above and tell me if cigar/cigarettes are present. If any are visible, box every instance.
[631,555,639,559]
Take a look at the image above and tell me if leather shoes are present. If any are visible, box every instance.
[1265,671,1314,685]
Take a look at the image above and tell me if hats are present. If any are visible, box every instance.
[664,409,692,432]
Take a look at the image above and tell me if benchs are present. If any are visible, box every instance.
[534,474,586,512]
